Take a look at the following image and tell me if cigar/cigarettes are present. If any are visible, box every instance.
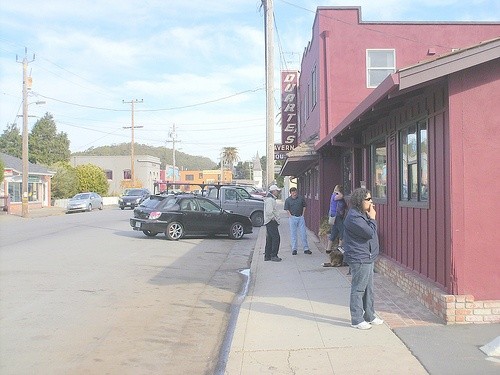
[373,205,375,207]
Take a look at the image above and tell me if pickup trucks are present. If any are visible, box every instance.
[178,184,265,228]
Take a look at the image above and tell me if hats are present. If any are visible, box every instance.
[269,184,281,191]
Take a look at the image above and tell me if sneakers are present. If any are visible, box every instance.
[367,317,383,327]
[351,321,372,330]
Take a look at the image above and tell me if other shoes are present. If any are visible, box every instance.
[304,250,312,255]
[264,255,271,261]
[326,249,332,254]
[291,250,297,255]
[271,256,282,262]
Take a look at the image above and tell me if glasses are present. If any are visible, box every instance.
[363,197,372,201]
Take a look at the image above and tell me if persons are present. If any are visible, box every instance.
[284,187,312,255]
[264,185,282,261]
[344,188,384,330]
[326,185,349,253]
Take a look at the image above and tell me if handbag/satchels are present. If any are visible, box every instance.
[328,216,336,226]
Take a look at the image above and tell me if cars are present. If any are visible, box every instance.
[119,189,151,210]
[130,192,253,241]
[67,192,103,214]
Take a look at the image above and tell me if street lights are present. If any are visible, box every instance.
[21,100,46,218]
